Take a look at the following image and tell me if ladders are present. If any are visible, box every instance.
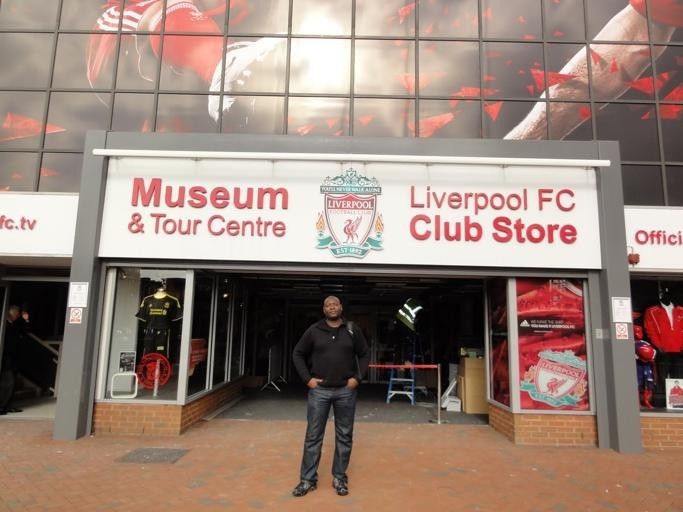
[384,370,415,405]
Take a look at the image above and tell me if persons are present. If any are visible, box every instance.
[633,325,656,409]
[87,0,288,132]
[135,289,183,361]
[0,304,32,415]
[503,0,683,141]
[644,288,683,393]
[293,296,371,496]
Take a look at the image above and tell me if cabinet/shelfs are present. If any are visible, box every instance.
[455,356,490,415]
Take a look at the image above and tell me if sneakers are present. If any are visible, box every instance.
[292,479,318,498]
[332,478,349,496]
